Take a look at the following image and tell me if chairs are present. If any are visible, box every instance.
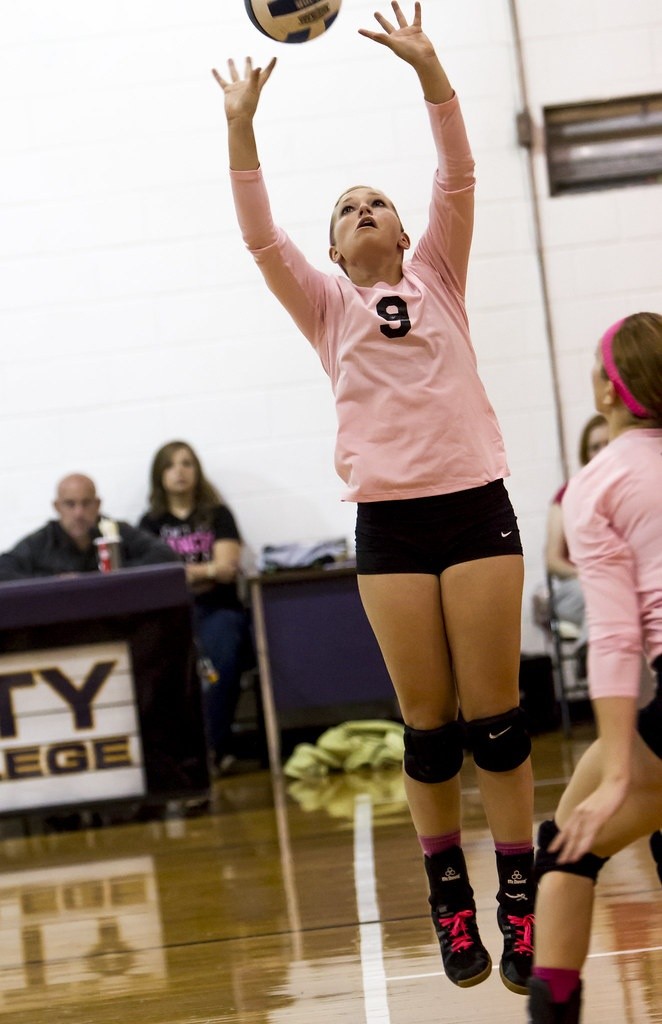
[546,572,589,740]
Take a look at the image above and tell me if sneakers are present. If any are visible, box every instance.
[429,899,493,988]
[497,904,538,995]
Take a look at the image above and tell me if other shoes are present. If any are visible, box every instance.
[210,751,243,778]
[182,794,211,818]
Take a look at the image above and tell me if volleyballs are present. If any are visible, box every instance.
[244,0,342,44]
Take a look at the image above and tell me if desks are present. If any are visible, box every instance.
[0,560,404,835]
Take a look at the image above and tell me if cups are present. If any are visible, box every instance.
[92,538,123,572]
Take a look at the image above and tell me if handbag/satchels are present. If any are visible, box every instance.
[259,536,346,582]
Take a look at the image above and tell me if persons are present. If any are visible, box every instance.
[525,310,662,1024]
[213,0,538,997]
[0,474,177,581]
[135,441,242,773]
